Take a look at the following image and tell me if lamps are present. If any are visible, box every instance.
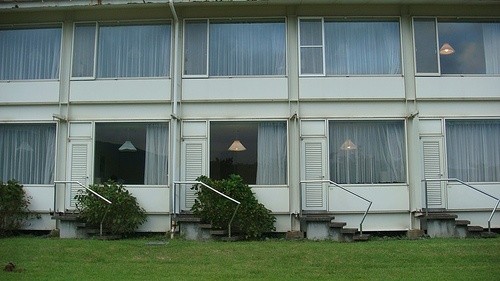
[228,139,246,150]
[119,140,136,152]
[339,139,357,151]
[440,41,455,55]
